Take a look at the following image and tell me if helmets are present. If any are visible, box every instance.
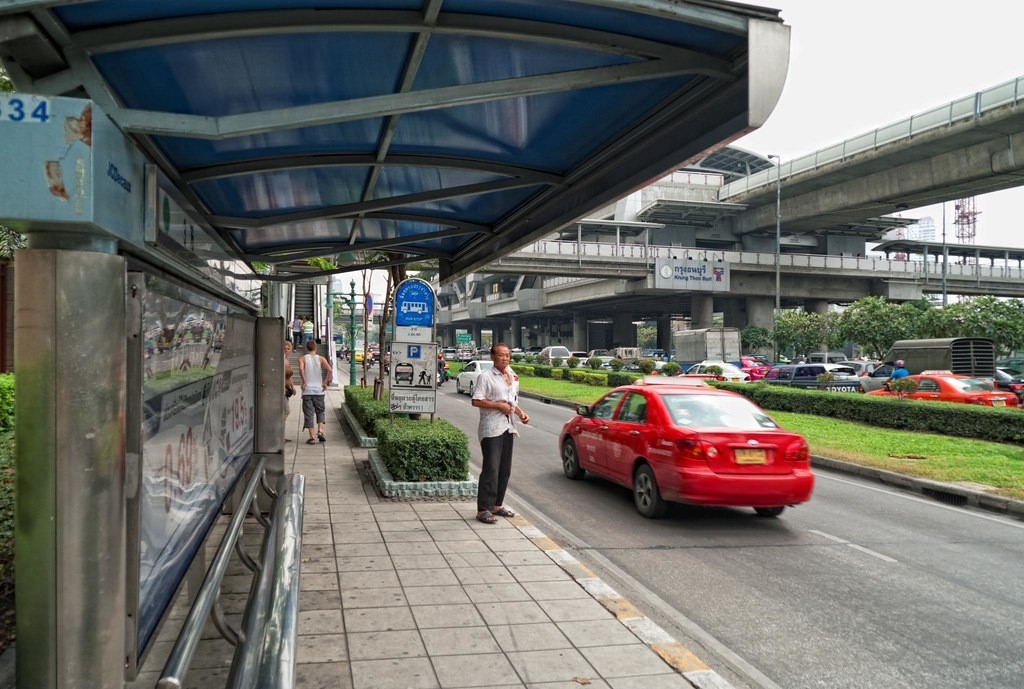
[896,360,905,367]
[438,354,442,359]
[386,352,390,356]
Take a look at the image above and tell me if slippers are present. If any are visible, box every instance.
[476,512,498,522]
[492,507,514,517]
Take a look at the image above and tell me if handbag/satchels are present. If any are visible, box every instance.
[286,385,293,397]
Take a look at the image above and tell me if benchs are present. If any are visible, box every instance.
[155,457,305,688]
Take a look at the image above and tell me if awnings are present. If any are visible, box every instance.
[0,0,795,290]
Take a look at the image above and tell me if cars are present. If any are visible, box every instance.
[456,358,496,397]
[558,385,816,519]
[632,377,712,390]
[354,341,390,361]
[438,343,1024,414]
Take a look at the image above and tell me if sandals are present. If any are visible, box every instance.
[307,439,315,444]
[318,431,325,442]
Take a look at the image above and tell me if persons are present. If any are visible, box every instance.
[471,343,530,525]
[339,345,351,360]
[883,359,909,386]
[363,348,376,368]
[384,352,389,373]
[797,357,806,366]
[284,339,333,446]
[286,314,315,352]
[437,353,451,386]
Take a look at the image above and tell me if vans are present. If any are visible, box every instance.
[882,336,997,379]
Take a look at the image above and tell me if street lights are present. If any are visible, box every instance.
[767,153,789,342]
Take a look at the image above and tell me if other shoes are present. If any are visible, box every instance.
[299,345,303,347]
[294,350,296,352]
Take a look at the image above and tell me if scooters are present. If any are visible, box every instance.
[340,352,350,359]
[436,364,450,389]
[367,355,375,364]
[385,364,390,374]
[362,360,370,370]
